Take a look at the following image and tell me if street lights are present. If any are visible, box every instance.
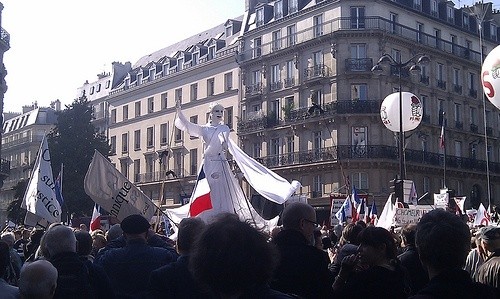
[369,51,433,204]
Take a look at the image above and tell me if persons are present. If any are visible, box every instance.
[174,103,299,232]
[0,200,500,299]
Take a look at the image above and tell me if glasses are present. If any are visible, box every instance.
[304,218,318,227]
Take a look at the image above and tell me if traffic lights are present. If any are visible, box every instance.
[389,177,402,202]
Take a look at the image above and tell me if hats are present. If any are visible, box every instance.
[480,225,500,240]
[120,214,151,234]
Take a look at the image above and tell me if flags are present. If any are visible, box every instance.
[334,185,378,227]
[20,136,61,224]
[54,170,64,205]
[88,203,102,232]
[439,120,445,147]
[189,165,214,218]
